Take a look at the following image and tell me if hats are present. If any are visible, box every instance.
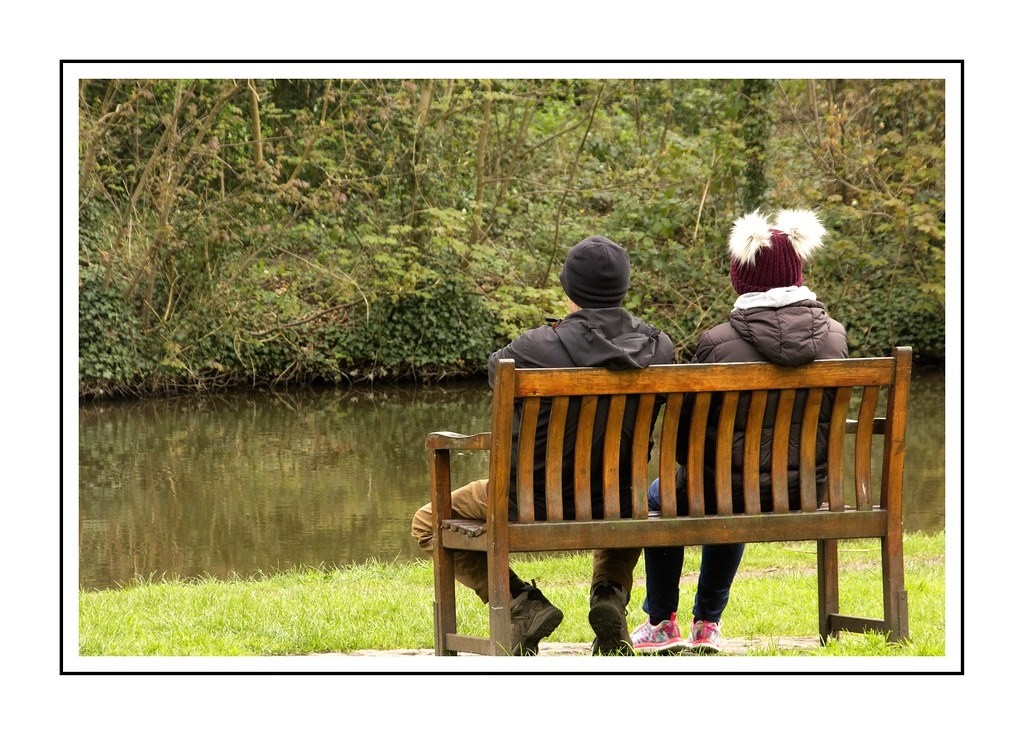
[727,204,827,296]
[559,235,631,308]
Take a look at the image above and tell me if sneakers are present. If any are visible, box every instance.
[628,612,685,653]
[682,617,724,652]
[586,580,635,656]
[509,578,563,656]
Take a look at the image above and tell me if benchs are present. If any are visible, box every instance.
[423,346,913,656]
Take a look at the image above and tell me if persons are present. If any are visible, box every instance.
[413,236,675,657]
[629,207,851,653]
